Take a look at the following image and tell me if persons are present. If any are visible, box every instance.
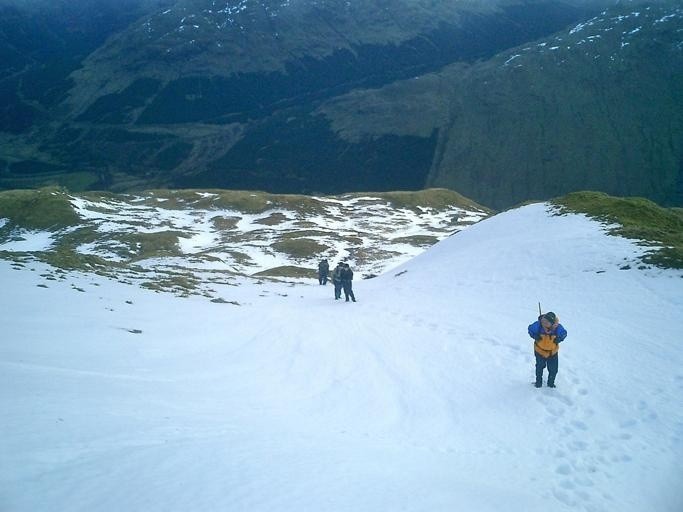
[340,264,355,302]
[318,258,328,285]
[331,262,343,300]
[527,311,567,388]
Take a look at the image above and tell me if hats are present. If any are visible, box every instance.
[339,262,349,268]
[544,312,555,324]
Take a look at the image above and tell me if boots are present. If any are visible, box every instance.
[548,375,556,387]
[535,377,542,387]
